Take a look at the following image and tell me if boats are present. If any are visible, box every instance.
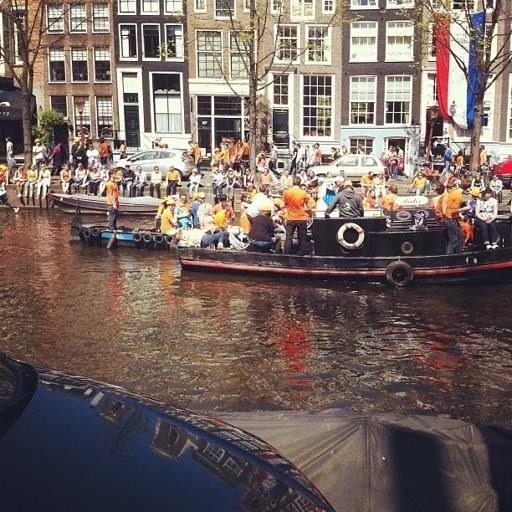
[48,193,165,217]
[72,219,180,251]
[180,208,512,287]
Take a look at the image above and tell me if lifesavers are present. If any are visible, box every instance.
[337,223,365,250]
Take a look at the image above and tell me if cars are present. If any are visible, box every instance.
[0,350,338,511]
[306,153,389,186]
[493,157,512,189]
[115,148,194,179]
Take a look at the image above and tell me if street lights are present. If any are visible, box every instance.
[75,97,87,168]
[425,104,441,164]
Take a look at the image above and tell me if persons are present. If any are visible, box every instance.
[1,128,512,258]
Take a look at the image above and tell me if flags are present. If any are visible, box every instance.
[430,11,488,132]
